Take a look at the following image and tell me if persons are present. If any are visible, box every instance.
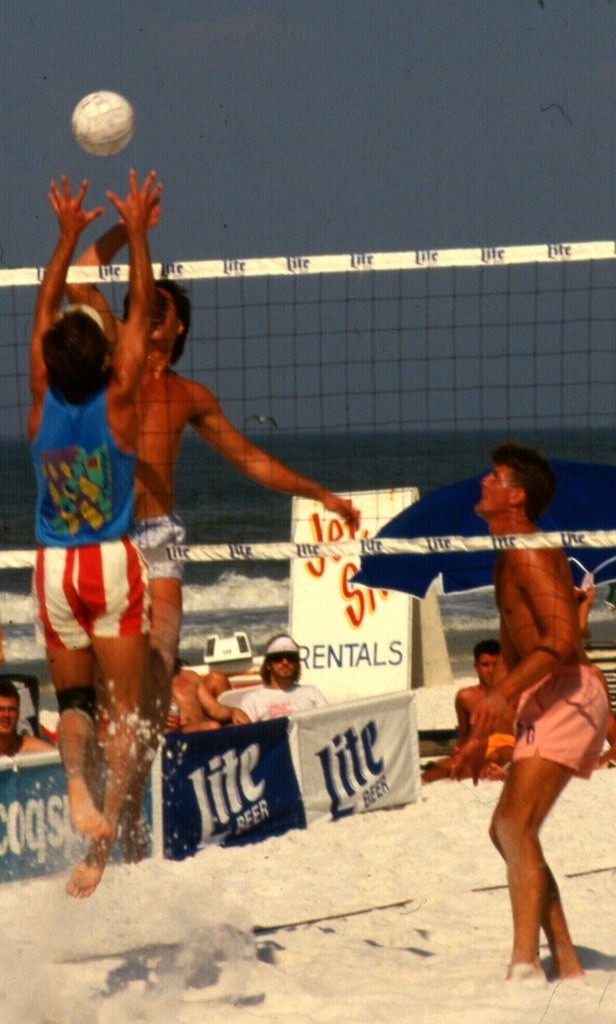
[63,191,364,865]
[163,658,249,734]
[240,629,333,725]
[0,682,55,757]
[421,638,514,784]
[449,445,616,993]
[27,166,168,896]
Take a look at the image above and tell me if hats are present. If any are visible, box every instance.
[265,636,299,654]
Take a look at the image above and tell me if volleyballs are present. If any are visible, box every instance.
[68,90,135,158]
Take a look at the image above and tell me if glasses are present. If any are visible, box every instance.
[270,652,298,663]
